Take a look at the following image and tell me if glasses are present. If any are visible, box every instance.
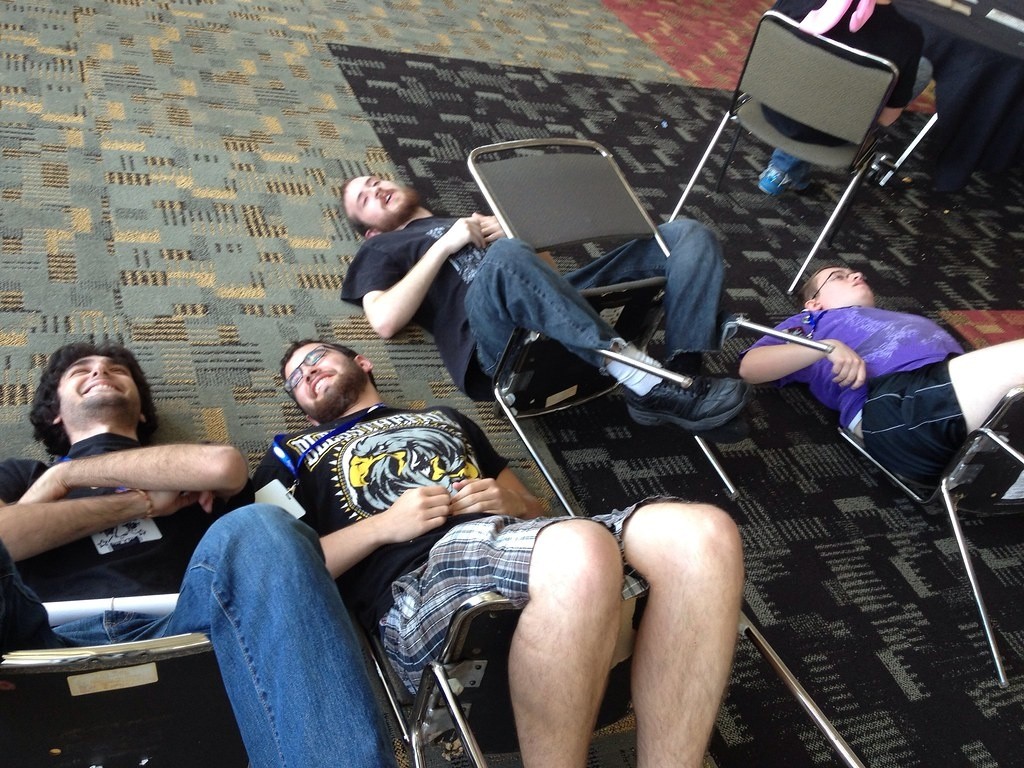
[283,344,344,410]
[812,270,866,299]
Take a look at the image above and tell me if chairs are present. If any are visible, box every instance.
[834,381,1024,686]
[0,632,420,768]
[464,140,834,521]
[291,496,865,768]
[665,11,902,297]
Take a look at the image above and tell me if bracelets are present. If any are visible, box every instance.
[134,487,153,520]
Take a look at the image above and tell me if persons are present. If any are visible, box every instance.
[0,341,396,768]
[338,174,749,432]
[245,337,745,768]
[734,262,1024,481]
[753,0,933,197]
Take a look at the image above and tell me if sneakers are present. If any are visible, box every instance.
[624,372,752,432]
[758,167,811,197]
